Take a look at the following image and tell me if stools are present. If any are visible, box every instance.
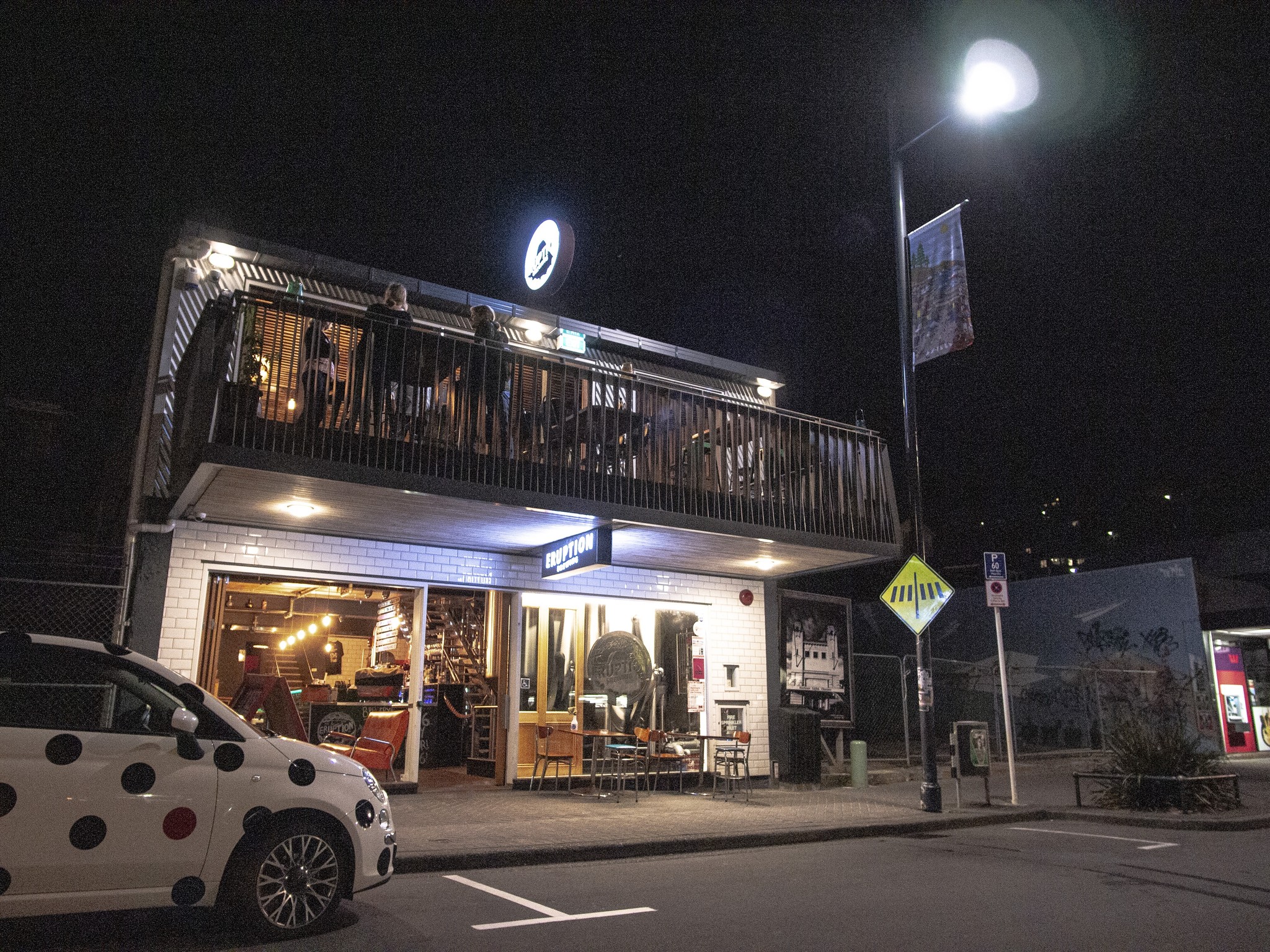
[598,744,638,803]
[713,747,748,801]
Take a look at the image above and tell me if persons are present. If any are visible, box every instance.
[341,332,512,457]
[465,304,501,453]
[295,317,341,429]
[356,283,413,438]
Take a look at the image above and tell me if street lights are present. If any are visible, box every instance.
[891,38,1038,812]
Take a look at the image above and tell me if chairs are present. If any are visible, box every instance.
[519,395,653,474]
[714,732,753,794]
[612,727,684,794]
[318,710,410,781]
[529,723,573,793]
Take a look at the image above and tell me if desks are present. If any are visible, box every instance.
[551,407,649,470]
[667,732,739,795]
[559,728,635,797]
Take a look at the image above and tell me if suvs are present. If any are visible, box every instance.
[0,632,397,942]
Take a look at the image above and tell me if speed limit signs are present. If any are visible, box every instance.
[984,552,1007,580]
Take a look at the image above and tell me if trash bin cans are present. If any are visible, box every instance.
[950,717,995,777]
[776,704,822,784]
[850,740,869,787]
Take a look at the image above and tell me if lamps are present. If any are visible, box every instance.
[279,584,332,650]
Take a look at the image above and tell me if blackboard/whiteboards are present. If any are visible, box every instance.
[227,672,308,741]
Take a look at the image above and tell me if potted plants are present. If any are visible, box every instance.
[224,302,269,416]
